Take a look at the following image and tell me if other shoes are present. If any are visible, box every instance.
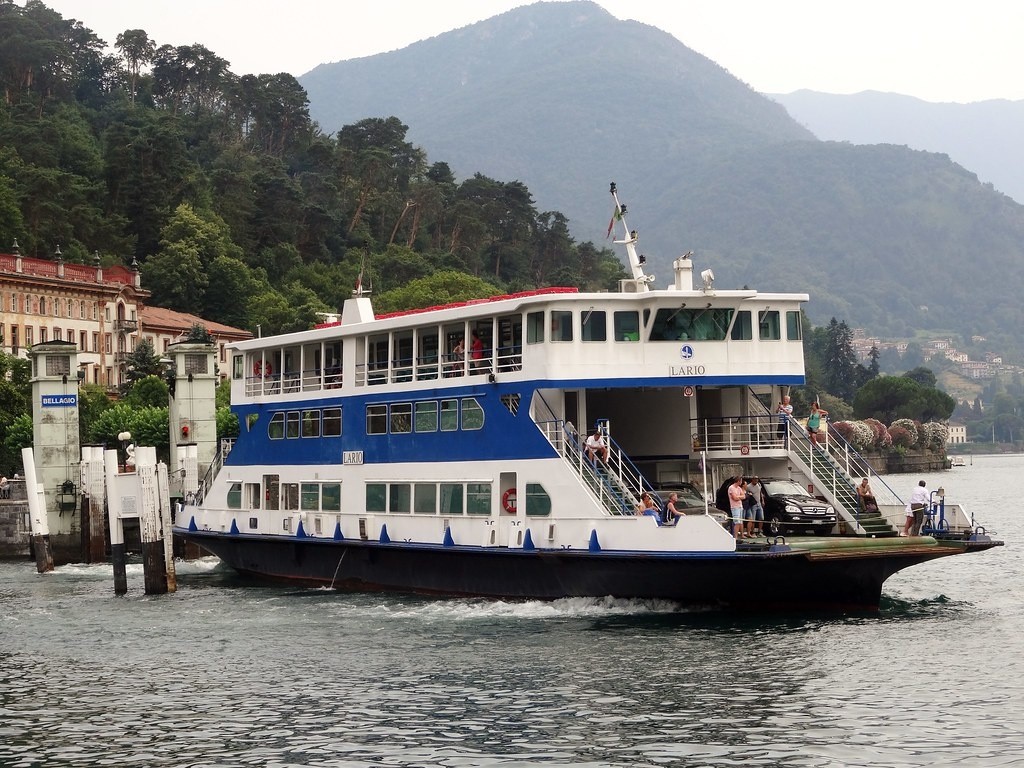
[746,534,752,538]
[603,464,609,469]
[757,532,767,538]
[751,532,758,538]
[588,464,594,469]
[741,536,747,539]
[900,532,910,536]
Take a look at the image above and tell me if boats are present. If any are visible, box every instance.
[949,456,966,467]
[169,179,1008,626]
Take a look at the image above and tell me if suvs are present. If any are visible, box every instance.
[715,475,837,536]
[647,483,729,530]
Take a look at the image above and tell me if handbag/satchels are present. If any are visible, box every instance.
[742,496,758,510]
[866,503,876,512]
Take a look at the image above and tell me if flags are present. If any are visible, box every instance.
[607,207,620,238]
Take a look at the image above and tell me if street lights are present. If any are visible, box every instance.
[117,431,132,472]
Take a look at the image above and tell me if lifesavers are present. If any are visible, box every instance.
[741,446,749,455]
[692,433,700,440]
[254,359,272,379]
[503,488,517,513]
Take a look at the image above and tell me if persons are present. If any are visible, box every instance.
[471,331,482,374]
[746,476,767,538]
[639,492,658,512]
[857,478,880,512]
[911,480,939,536]
[328,365,340,388]
[900,503,914,536]
[741,481,753,538]
[661,492,685,525]
[728,476,747,539]
[452,337,464,376]
[583,431,610,469]
[776,396,793,448]
[0,474,8,486]
[806,402,828,443]
